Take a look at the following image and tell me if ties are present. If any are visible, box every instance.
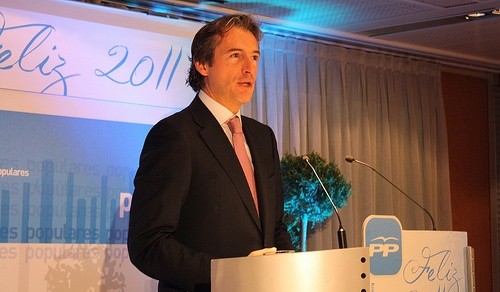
[226,118,259,215]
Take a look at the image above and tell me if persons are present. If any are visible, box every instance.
[127,13,294,292]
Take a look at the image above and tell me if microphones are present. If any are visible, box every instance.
[302,155,347,249]
[344,155,436,231]
